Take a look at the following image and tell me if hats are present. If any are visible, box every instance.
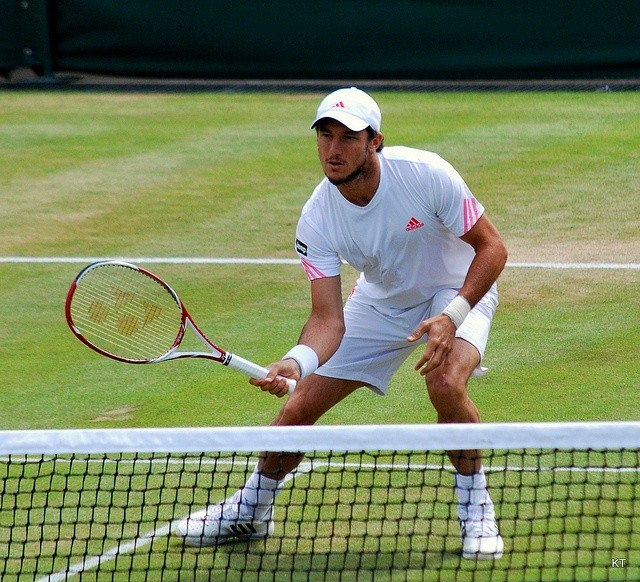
[311,87,382,133]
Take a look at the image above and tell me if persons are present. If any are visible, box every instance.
[176,86,507,562]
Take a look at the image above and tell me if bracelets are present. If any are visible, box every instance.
[441,294,472,329]
[282,343,320,382]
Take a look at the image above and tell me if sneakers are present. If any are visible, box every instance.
[176,492,274,547]
[458,500,504,560]
